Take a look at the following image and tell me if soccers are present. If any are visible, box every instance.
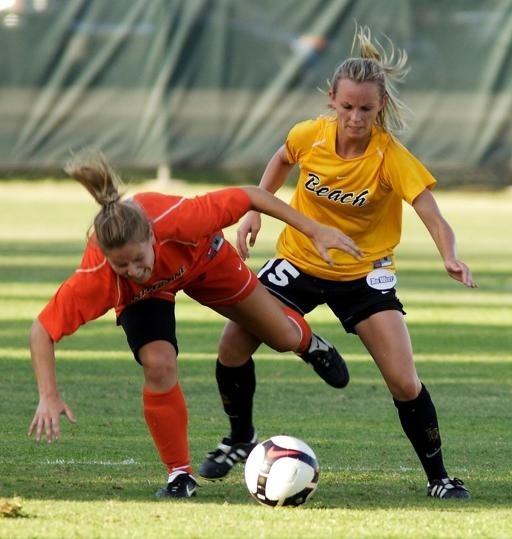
[245,435,320,508]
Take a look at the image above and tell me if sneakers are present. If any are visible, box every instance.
[426,478,473,501]
[153,472,199,501]
[198,431,259,481]
[295,333,351,391]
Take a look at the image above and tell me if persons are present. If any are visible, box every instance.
[26,143,369,503]
[196,16,481,500]
